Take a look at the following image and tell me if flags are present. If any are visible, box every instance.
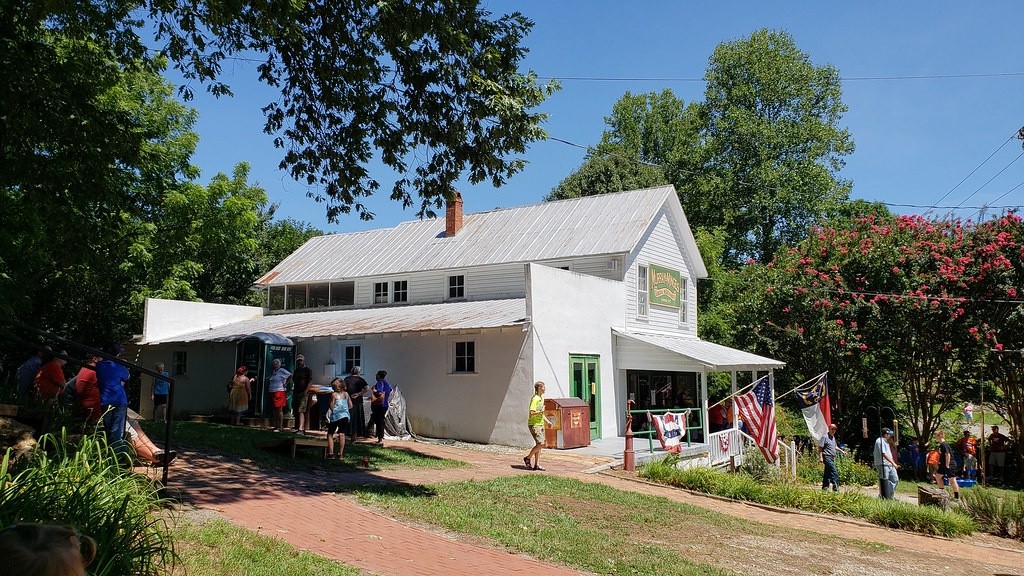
[734,377,780,464]
[797,375,831,441]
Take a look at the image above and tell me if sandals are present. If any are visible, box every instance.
[534,466,546,471]
[524,456,532,469]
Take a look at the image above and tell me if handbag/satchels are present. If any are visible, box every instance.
[226,374,235,394]
[371,381,385,404]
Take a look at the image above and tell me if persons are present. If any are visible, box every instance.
[709,397,747,446]
[819,424,848,491]
[269,358,293,431]
[524,382,552,471]
[367,370,391,445]
[151,363,171,422]
[690,417,699,441]
[18,344,177,468]
[292,355,313,435]
[988,426,1012,483]
[674,397,684,412]
[229,367,257,425]
[909,431,978,503]
[964,403,973,424]
[631,414,655,439]
[874,428,899,494]
[327,378,353,459]
[343,367,370,442]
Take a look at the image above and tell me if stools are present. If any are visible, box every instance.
[292,438,331,460]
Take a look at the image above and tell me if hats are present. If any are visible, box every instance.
[963,431,970,434]
[991,426,999,429]
[882,428,893,434]
[295,354,305,361]
[829,424,837,428]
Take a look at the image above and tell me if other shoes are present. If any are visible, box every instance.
[237,423,244,426]
[351,433,357,441]
[328,454,335,459]
[153,418,158,422]
[278,428,285,432]
[231,422,234,425]
[295,430,305,436]
[163,419,168,423]
[338,455,344,461]
[375,441,384,446]
